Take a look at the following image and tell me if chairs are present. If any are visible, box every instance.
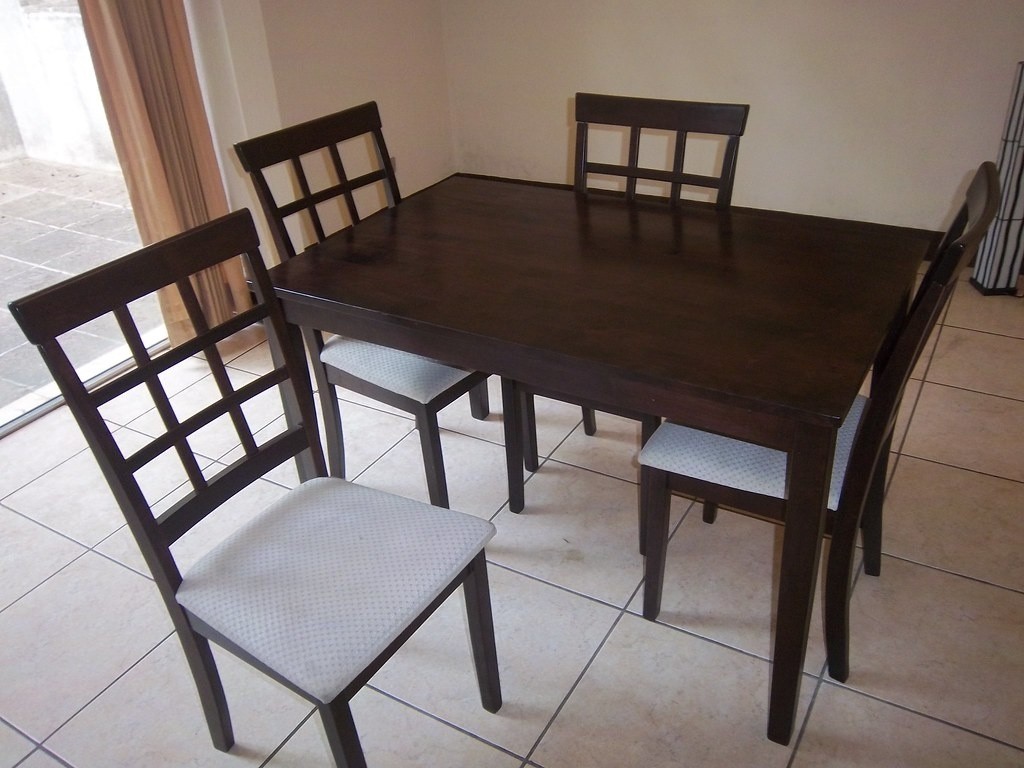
[638,161,999,683]
[518,92,750,472]
[7,207,503,768]
[234,101,493,509]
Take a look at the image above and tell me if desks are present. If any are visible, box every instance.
[249,171,933,747]
